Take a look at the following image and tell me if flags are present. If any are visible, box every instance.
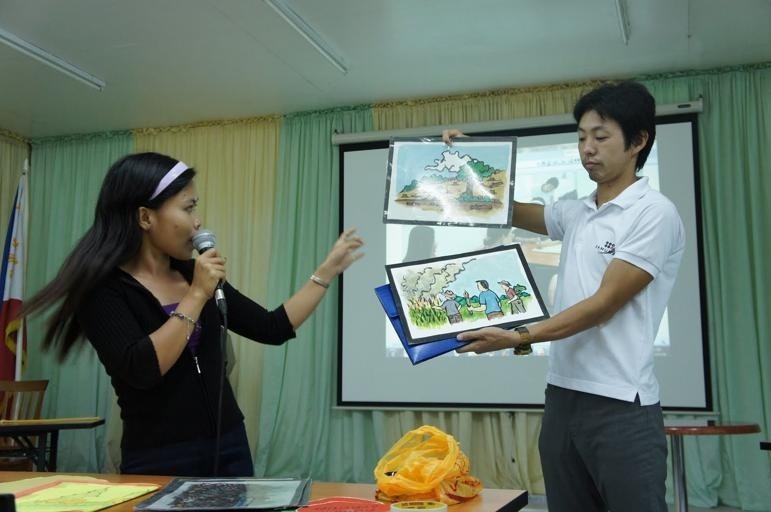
[0,154,28,421]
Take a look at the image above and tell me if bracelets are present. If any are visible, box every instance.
[309,274,330,290]
[170,309,197,340]
[513,325,533,356]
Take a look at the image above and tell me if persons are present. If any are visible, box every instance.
[390,175,562,324]
[438,81,688,511]
[13,149,366,482]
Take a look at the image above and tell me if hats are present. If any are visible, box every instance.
[442,291,457,298]
[497,281,510,286]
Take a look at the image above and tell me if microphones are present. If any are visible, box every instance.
[190,230,227,317]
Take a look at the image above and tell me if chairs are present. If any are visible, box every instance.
[0,380,49,470]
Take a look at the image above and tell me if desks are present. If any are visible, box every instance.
[0,417,106,472]
[0,474,528,511]
[664,426,761,512]
